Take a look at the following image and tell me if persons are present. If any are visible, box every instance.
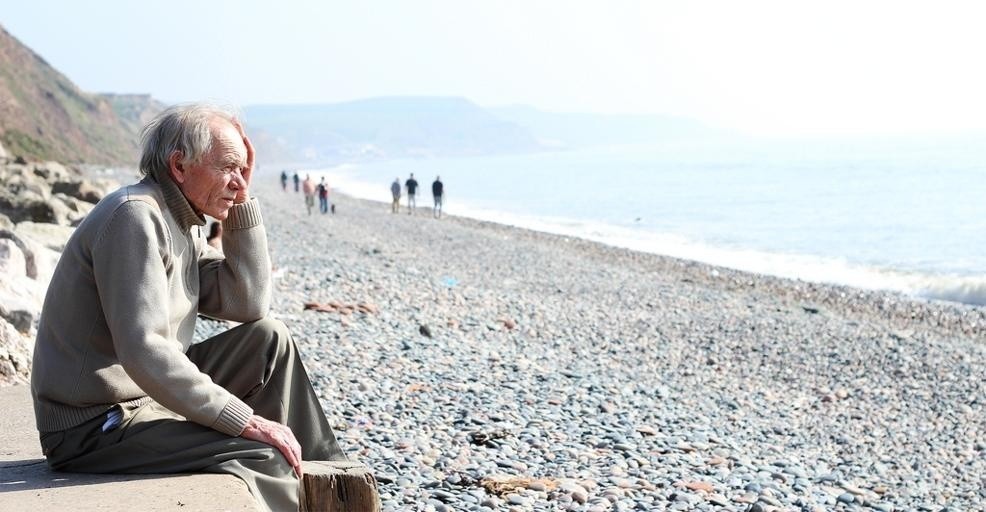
[315,175,328,214]
[301,173,317,215]
[389,178,401,215]
[292,172,299,192]
[403,171,422,217]
[429,175,444,220]
[27,101,351,511]
[203,220,226,255]
[279,169,289,191]
